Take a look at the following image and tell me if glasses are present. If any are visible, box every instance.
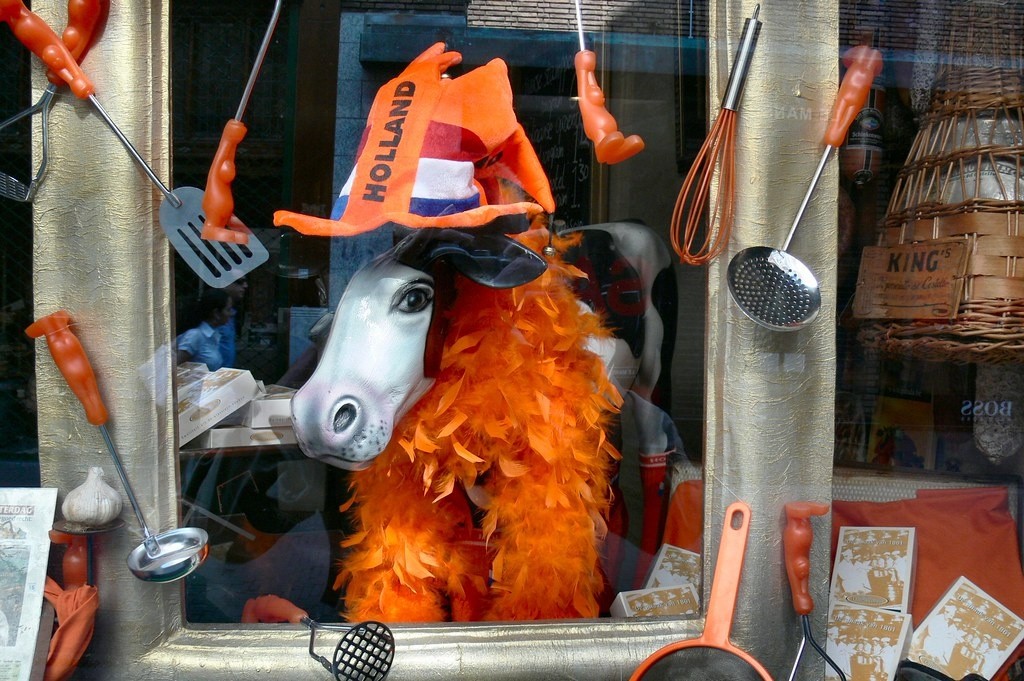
[233,277,249,287]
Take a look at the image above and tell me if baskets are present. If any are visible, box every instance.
[857,70,1024,362]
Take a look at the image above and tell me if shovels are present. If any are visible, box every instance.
[1,0,274,292]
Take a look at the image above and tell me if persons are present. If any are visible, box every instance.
[177,267,250,494]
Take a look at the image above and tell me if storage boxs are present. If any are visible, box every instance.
[176,362,299,445]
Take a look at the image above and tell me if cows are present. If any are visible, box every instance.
[290,221,680,547]
